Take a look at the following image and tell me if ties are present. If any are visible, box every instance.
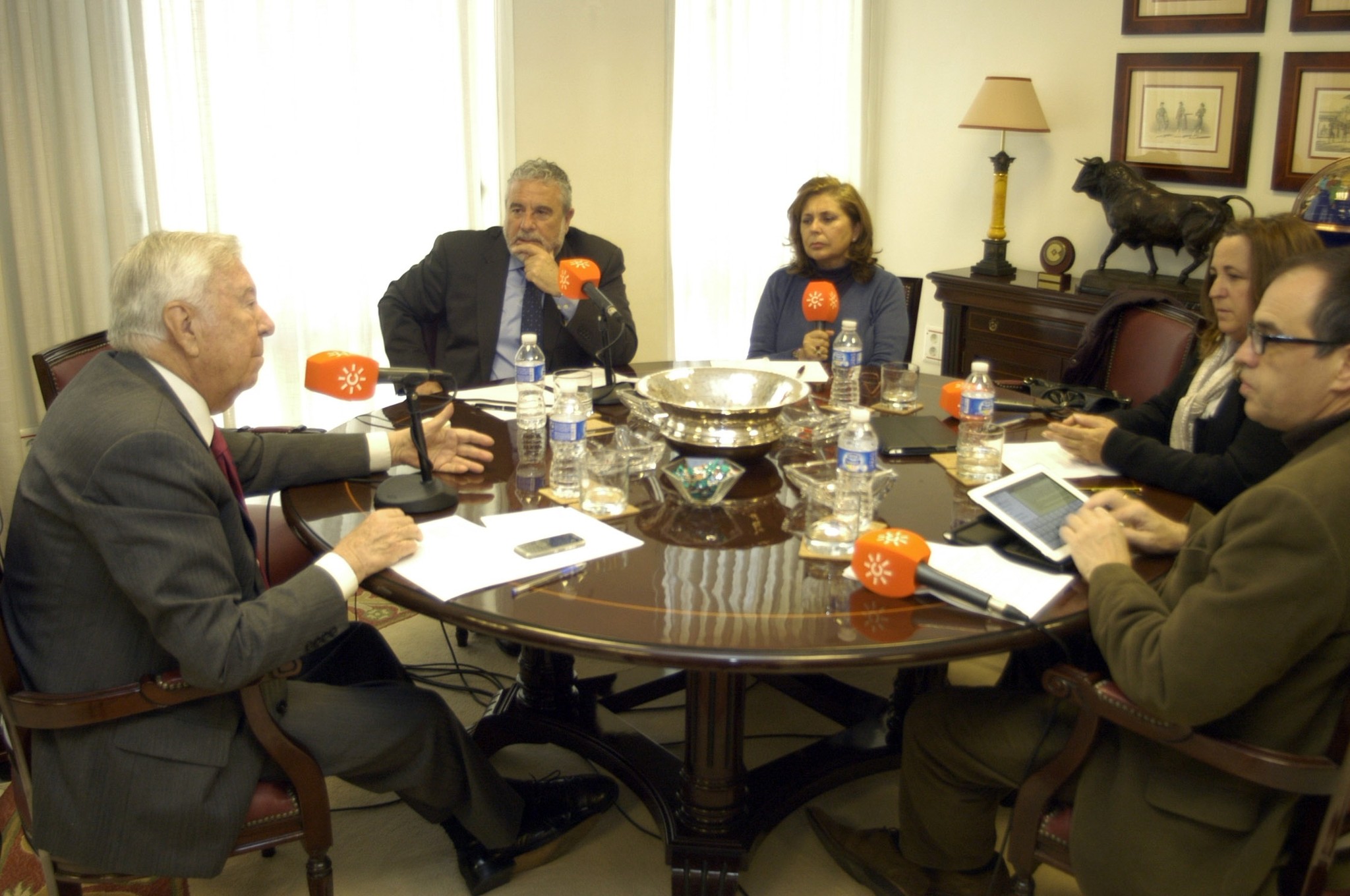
[210,423,269,591]
[521,280,543,351]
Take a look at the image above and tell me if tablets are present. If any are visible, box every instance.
[966,461,1090,561]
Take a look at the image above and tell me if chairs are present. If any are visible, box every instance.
[992,294,1210,416]
[0,608,334,896]
[1000,661,1350,896]
[32,331,327,589]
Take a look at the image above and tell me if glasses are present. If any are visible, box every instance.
[1246,322,1350,355]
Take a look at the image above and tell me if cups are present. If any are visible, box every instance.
[579,449,629,514]
[879,361,918,406]
[560,369,593,417]
[956,421,1006,485]
[805,481,860,554]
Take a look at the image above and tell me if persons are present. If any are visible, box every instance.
[379,157,637,404]
[805,214,1348,896]
[748,176,910,368]
[0,231,621,896]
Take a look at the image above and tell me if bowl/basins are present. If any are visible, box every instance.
[633,368,812,457]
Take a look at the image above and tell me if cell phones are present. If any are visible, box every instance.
[514,533,586,558]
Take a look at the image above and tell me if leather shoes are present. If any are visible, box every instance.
[456,811,579,896]
[508,766,619,816]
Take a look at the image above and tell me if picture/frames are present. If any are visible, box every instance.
[1110,51,1260,188]
[1289,0,1350,32]
[1272,51,1350,192]
[1120,0,1267,34]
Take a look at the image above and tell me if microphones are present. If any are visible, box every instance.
[850,528,1030,621]
[557,258,626,325]
[304,350,452,401]
[802,282,840,331]
[938,380,1042,421]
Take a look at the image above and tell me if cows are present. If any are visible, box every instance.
[1072,156,1255,287]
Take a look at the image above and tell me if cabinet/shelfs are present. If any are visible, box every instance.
[927,266,1101,389]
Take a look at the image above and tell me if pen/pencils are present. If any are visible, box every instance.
[475,403,516,412]
[997,417,1026,428]
[795,366,805,380]
[1078,487,1142,493]
[512,563,588,597]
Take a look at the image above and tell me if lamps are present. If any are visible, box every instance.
[958,77,1051,279]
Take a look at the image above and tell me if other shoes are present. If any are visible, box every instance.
[934,849,1011,896]
[806,807,930,896]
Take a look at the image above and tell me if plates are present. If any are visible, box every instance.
[710,358,828,387]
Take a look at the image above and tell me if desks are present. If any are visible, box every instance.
[282,360,1177,896]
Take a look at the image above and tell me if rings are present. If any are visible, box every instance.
[816,346,820,355]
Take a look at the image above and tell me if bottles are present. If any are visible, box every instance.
[834,407,875,532]
[516,427,546,510]
[829,320,862,409]
[548,383,589,498]
[957,361,995,453]
[515,333,546,429]
